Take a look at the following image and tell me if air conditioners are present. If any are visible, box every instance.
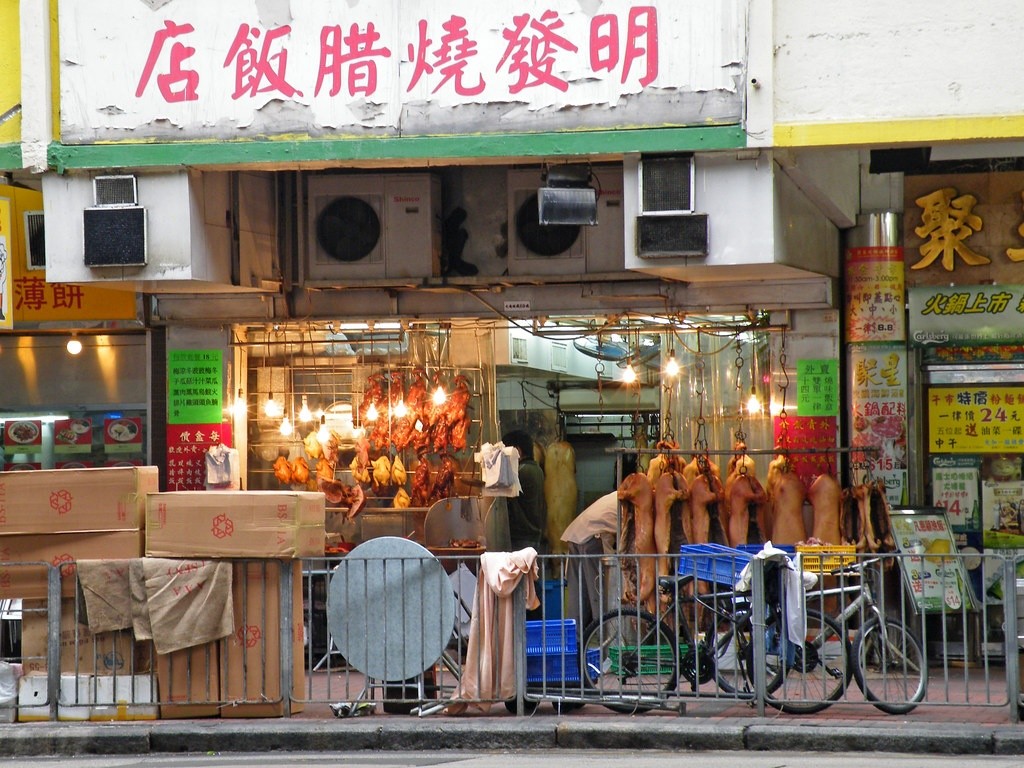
[506,163,633,276]
[307,173,444,280]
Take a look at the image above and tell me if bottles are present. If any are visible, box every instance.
[988,559,1024,600]
[971,499,979,529]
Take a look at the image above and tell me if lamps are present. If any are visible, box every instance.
[278,334,292,436]
[235,346,247,416]
[351,416,364,439]
[316,381,330,445]
[432,327,447,405]
[622,327,636,384]
[299,341,312,423]
[365,332,379,422]
[393,339,407,417]
[746,340,761,414]
[67,332,83,354]
[664,333,679,377]
[265,333,277,417]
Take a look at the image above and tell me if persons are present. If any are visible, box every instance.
[560,490,630,640]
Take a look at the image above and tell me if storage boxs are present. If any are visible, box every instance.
[0,531,143,600]
[1,465,158,535]
[20,600,134,678]
[798,545,858,572]
[527,649,601,686]
[152,640,218,719]
[608,645,689,674]
[527,620,578,655]
[219,561,306,718]
[144,491,324,556]
[16,674,158,721]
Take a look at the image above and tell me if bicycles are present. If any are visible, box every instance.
[704,546,931,713]
[576,551,854,719]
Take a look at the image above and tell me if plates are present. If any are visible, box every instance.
[69,419,90,434]
[108,419,139,442]
[7,421,41,444]
